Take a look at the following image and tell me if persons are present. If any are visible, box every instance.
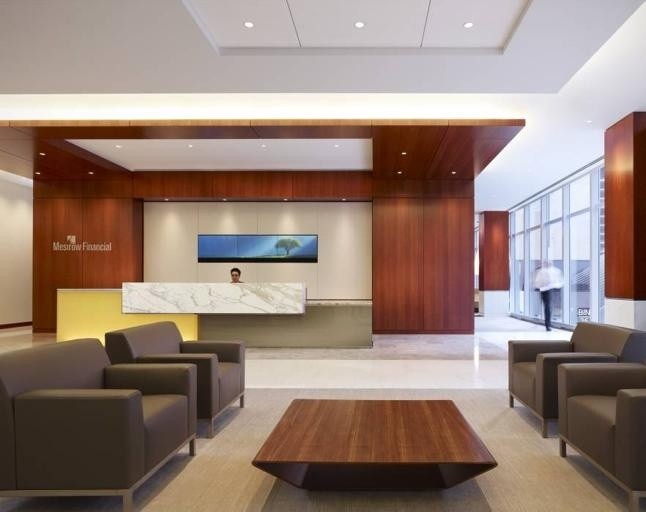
[230,268,244,283]
[533,257,563,331]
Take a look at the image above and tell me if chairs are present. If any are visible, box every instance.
[3,336,196,507]
[508,320,646,512]
[102,322,245,439]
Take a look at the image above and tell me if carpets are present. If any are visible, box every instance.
[0,387,646,512]
